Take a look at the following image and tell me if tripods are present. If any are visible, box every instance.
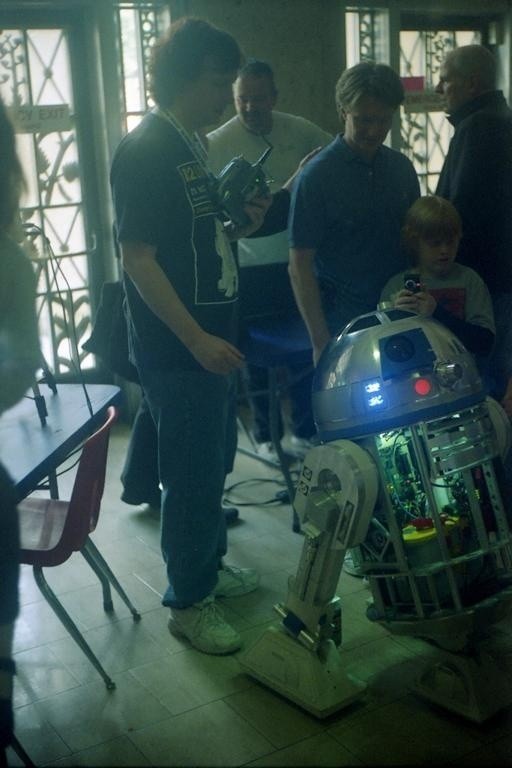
[31,348,58,426]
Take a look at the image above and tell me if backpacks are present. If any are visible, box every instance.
[81,280,139,387]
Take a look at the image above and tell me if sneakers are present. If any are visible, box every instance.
[167,595,242,655]
[290,434,323,456]
[204,566,262,597]
[342,549,366,577]
[121,484,163,506]
[258,441,290,467]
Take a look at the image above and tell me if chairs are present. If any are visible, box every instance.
[16,405,143,692]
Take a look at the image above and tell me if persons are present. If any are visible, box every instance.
[377,197,493,361]
[432,40,511,406]
[284,59,420,368]
[108,10,275,659]
[0,95,42,767]
[204,59,335,465]
[118,390,239,525]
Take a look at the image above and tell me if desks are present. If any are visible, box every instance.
[0,382,123,613]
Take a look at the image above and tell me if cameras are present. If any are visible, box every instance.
[403,273,421,293]
